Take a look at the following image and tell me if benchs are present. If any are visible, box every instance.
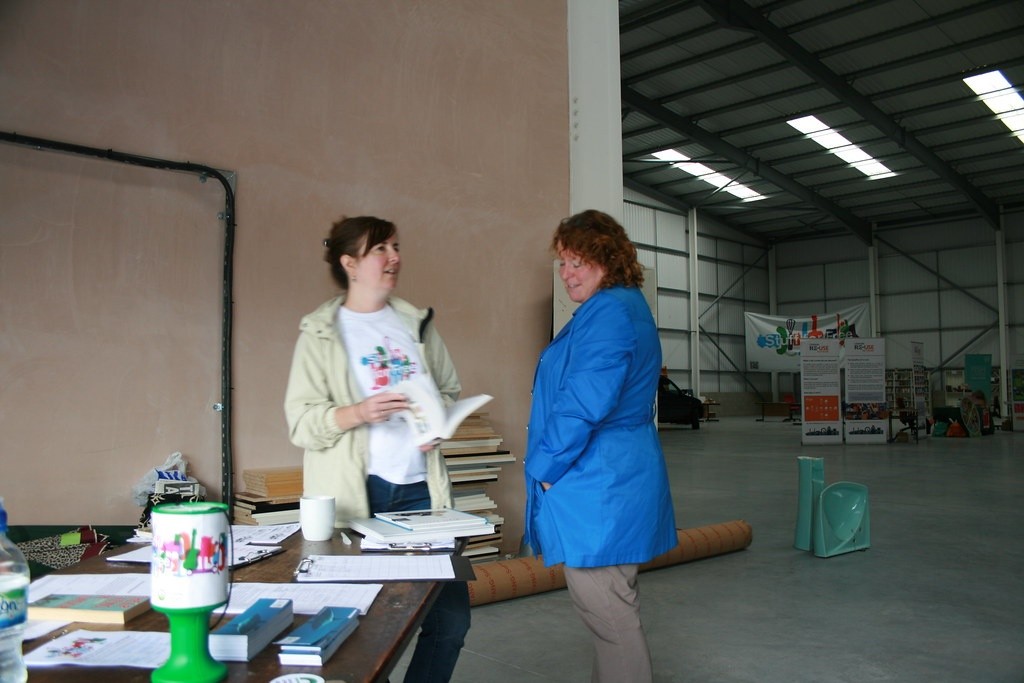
[753,401,801,422]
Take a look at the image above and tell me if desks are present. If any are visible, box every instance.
[888,408,921,444]
[0,535,466,683]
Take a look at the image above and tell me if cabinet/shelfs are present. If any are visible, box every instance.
[941,367,973,409]
[883,368,933,430]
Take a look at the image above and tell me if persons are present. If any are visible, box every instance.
[282,219,474,683]
[523,209,678,683]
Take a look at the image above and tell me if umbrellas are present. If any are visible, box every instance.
[15,525,115,571]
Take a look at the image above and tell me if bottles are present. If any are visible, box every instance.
[0,499,30,683]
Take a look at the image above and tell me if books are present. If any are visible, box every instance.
[27,592,151,624]
[439,411,517,565]
[388,375,494,447]
[347,506,495,542]
[127,527,153,542]
[232,467,302,526]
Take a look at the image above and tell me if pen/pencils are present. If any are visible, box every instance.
[339,528,351,545]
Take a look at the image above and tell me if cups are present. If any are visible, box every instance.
[300,498,336,541]
